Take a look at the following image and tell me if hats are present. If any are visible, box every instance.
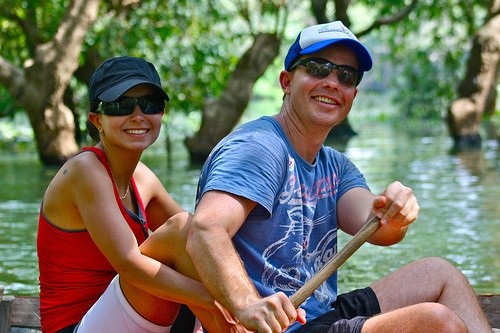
[284,19,373,73]
[89,55,170,113]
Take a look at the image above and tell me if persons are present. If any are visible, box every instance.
[184,19,494,332]
[37,52,259,332]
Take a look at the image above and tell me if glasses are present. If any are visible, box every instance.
[288,56,362,87]
[92,94,167,116]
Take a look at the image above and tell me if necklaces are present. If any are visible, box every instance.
[99,141,130,198]
